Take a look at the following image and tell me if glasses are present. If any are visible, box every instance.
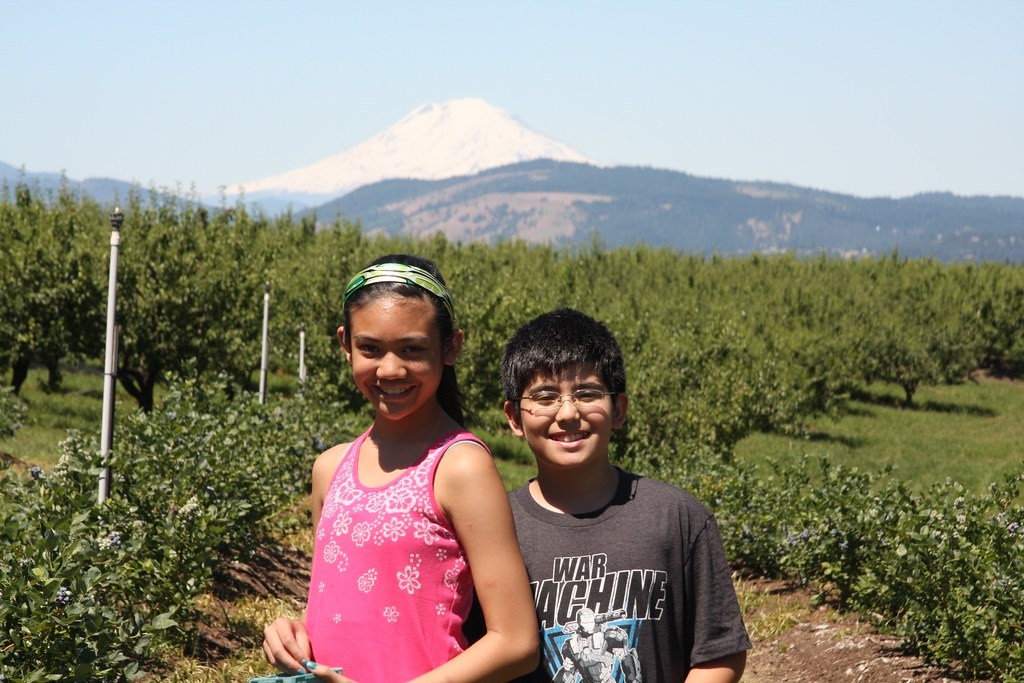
[511,388,615,409]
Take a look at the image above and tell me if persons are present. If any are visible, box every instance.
[460,307,752,683]
[261,253,540,683]
